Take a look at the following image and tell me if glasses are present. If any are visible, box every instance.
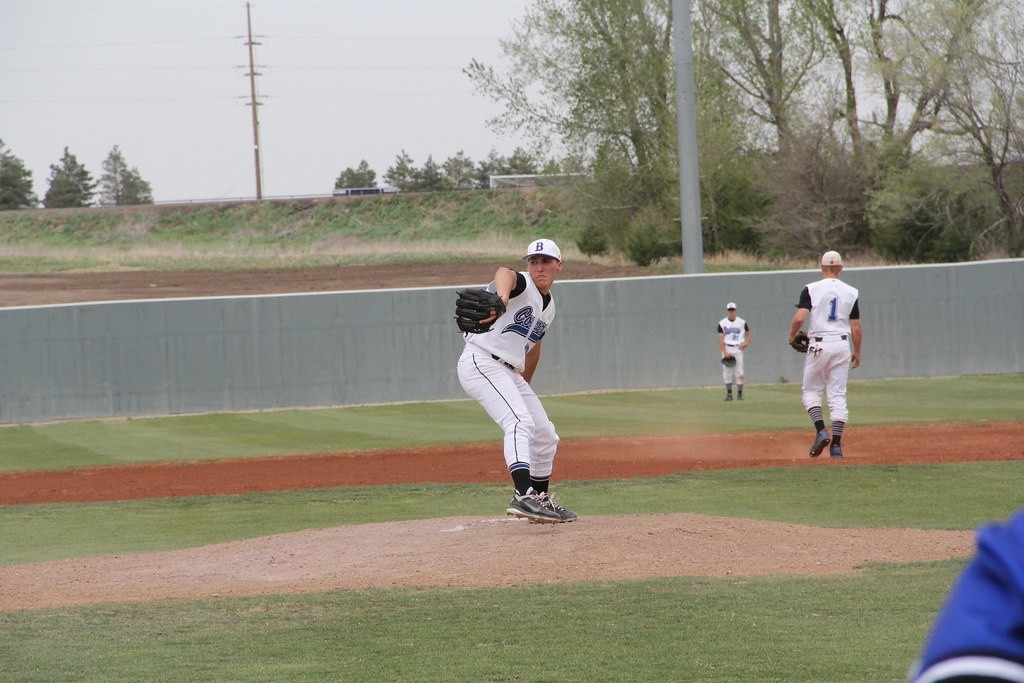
[728,308,734,311]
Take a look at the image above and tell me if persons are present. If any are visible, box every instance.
[788,250,861,458]
[717,302,751,401]
[911,510,1024,682]
[457,238,578,524]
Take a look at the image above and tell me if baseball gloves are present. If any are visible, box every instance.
[790,331,809,353]
[456,286,505,334]
[721,355,737,367]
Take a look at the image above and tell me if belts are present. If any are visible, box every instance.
[492,354,515,369]
[815,336,846,342]
[727,344,734,346]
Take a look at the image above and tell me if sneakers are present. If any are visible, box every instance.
[528,491,577,523]
[506,486,561,524]
[809,430,830,457]
[830,443,842,458]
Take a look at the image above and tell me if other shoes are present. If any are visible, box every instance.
[724,395,734,401]
[737,395,743,400]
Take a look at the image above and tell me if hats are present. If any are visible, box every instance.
[521,239,561,263]
[822,250,842,266]
[727,302,736,309]
